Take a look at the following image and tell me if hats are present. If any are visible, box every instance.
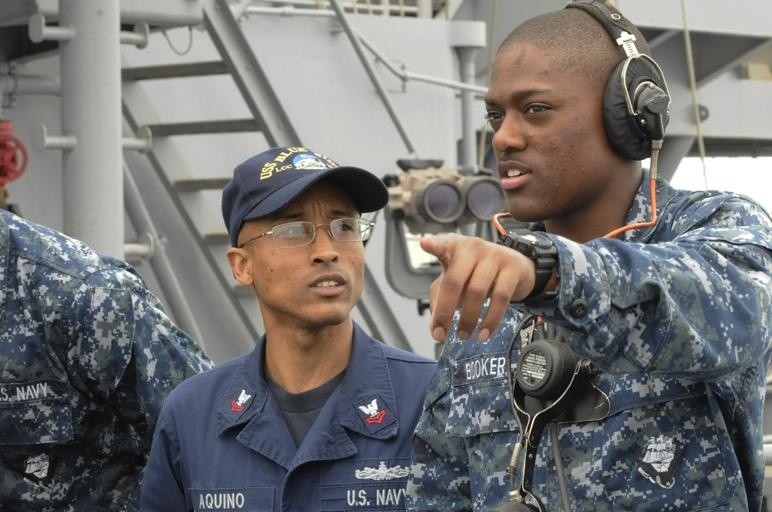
[219,144,389,249]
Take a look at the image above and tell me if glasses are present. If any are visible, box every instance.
[239,218,377,249]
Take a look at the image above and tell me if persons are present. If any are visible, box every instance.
[404,0,772,512]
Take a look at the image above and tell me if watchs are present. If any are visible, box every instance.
[497,228,558,304]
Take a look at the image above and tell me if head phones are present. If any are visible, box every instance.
[564,1,673,161]
